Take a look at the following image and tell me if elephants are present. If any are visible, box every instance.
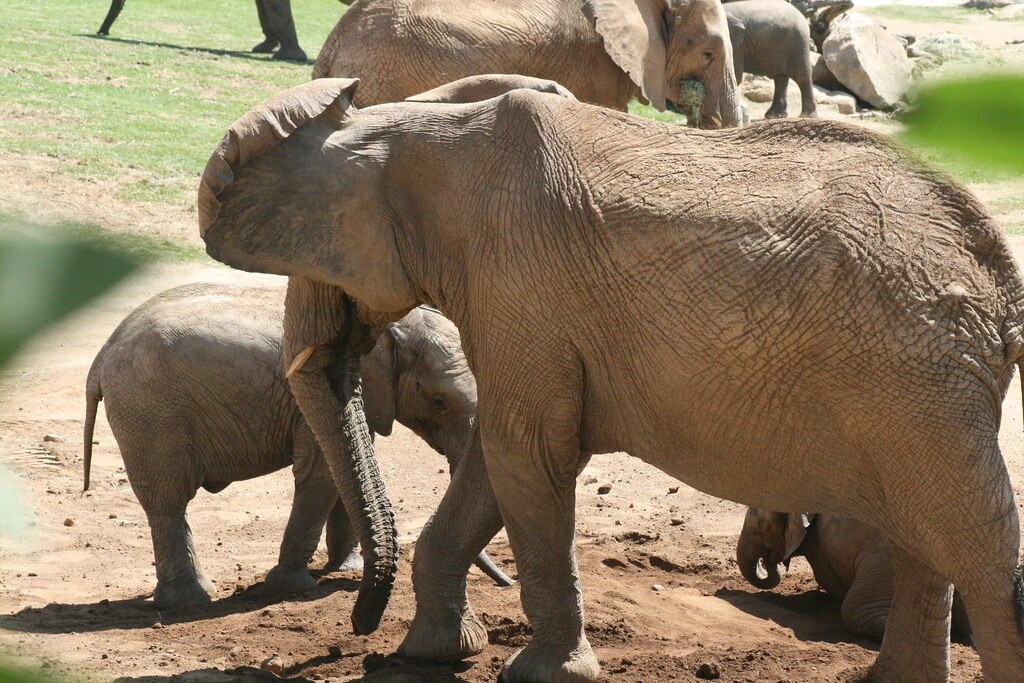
[80,0,1024,682]
